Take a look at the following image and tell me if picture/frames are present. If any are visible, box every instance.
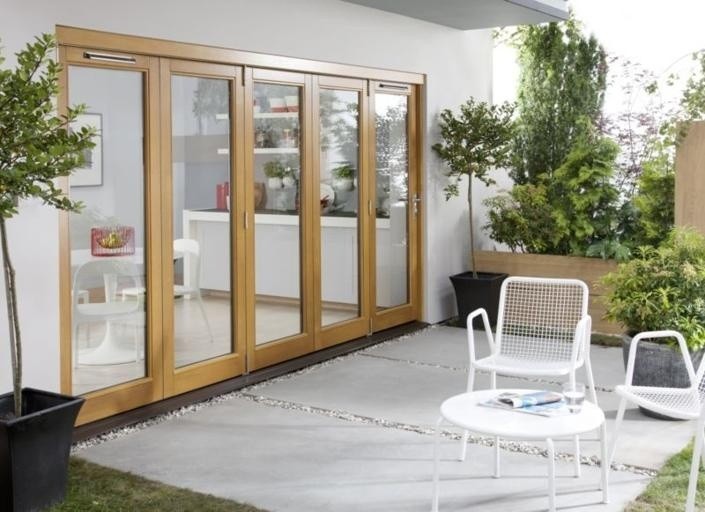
[68,112,104,187]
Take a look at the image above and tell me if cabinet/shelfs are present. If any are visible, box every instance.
[216,111,299,154]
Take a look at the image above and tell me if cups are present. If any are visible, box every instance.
[562,381,586,413]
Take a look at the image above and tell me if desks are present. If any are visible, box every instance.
[431,389,609,512]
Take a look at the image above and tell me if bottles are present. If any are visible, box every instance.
[256,131,268,149]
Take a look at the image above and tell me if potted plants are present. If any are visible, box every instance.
[592,223,705,421]
[0,31,103,512]
[261,161,296,189]
[431,95,523,330]
[330,166,353,191]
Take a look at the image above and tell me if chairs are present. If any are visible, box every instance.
[71,238,214,369]
[598,330,705,512]
[459,276,597,477]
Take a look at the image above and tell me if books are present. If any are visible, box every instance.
[480,387,582,420]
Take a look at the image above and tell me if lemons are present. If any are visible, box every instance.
[101,232,122,248]
